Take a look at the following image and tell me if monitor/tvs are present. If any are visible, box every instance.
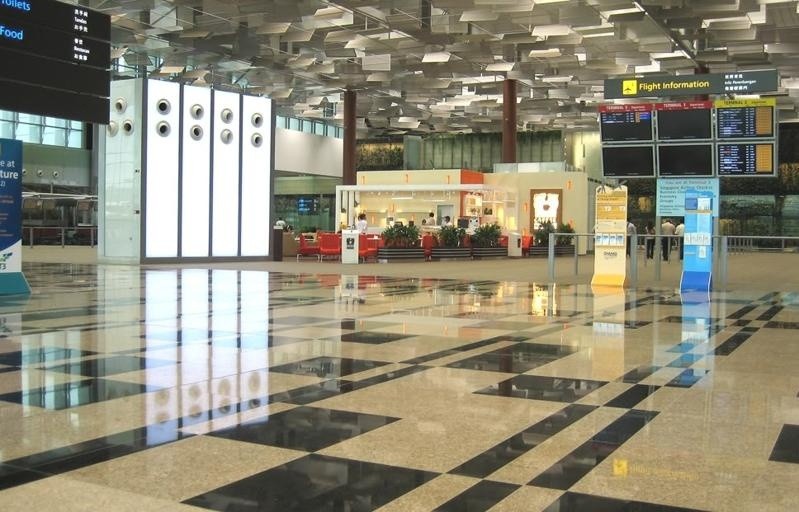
[457,218,468,228]
[394,221,402,225]
[409,221,414,227]
[598,106,779,178]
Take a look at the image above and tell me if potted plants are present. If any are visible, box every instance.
[529,220,576,256]
[377,224,509,261]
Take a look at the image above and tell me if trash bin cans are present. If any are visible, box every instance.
[507,232,522,259]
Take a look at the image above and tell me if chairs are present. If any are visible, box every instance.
[295,229,383,262]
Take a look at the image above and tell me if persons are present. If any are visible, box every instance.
[445,216,453,226]
[674,218,684,262]
[427,213,435,224]
[276,218,286,227]
[645,222,656,259]
[356,214,367,234]
[422,219,426,224]
[627,216,636,257]
[286,225,292,233]
[661,218,675,261]
